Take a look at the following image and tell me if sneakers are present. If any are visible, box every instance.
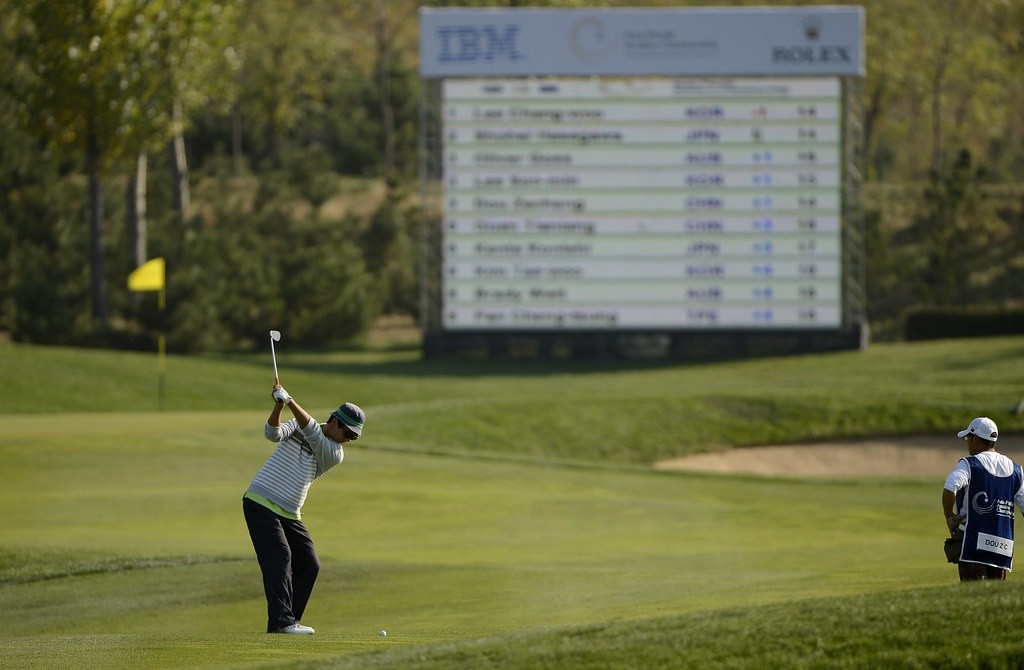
[273,621,314,634]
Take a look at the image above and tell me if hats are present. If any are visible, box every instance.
[330,401,366,437]
[957,417,999,443]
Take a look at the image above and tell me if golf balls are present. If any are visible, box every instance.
[380,631,386,636]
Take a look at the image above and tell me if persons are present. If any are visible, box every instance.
[242,385,366,634]
[942,417,1024,582]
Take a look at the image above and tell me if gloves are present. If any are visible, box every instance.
[274,386,291,404]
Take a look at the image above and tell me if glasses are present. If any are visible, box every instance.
[336,420,359,440]
[964,434,972,441]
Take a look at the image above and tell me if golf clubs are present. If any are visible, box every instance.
[270,331,284,385]
